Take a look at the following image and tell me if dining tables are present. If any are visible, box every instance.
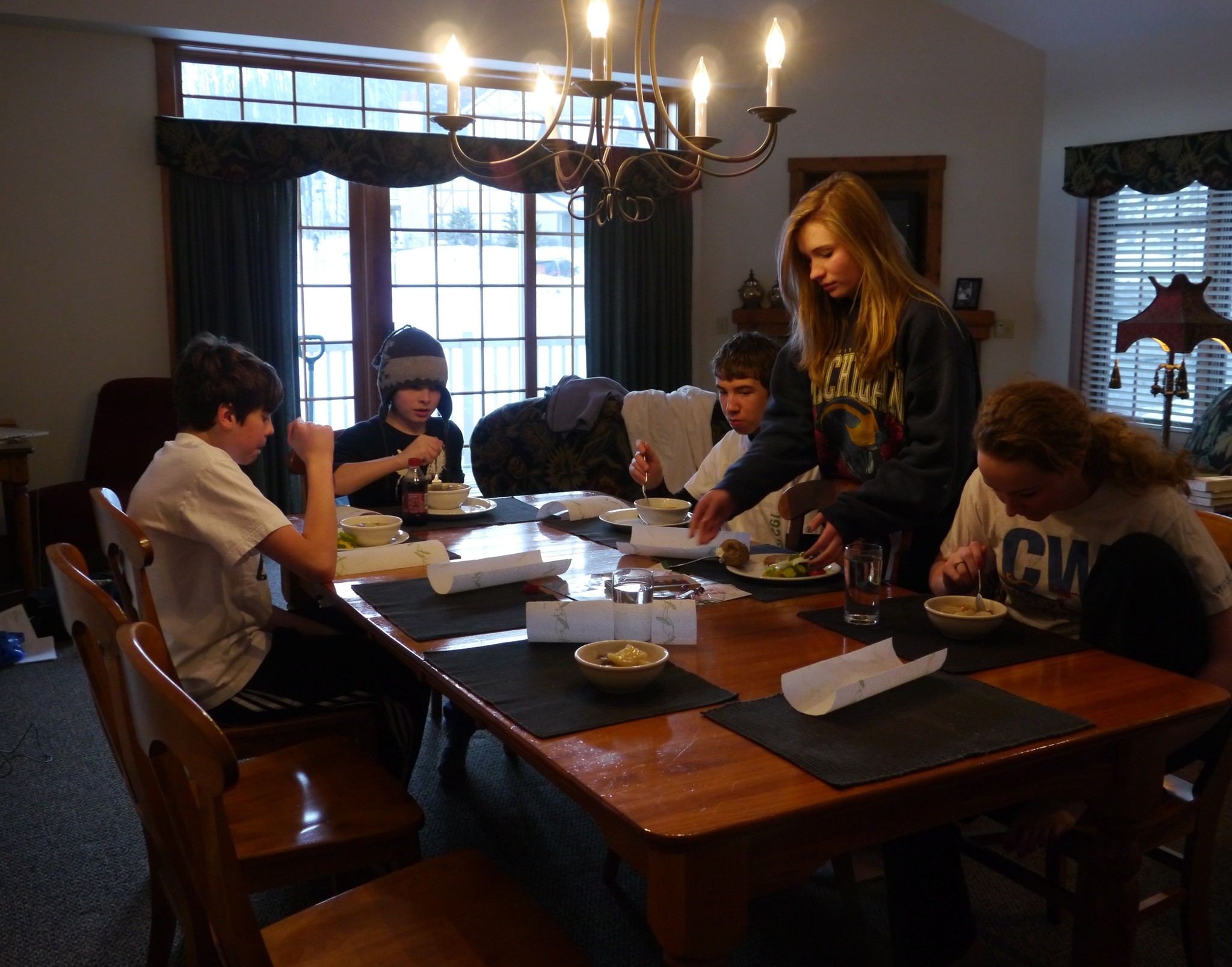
[263,489,1232,967]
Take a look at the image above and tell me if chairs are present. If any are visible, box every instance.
[285,428,442,718]
[44,540,426,967]
[1184,385,1232,477]
[960,509,1232,967]
[28,377,179,583]
[87,485,377,760]
[113,619,594,967]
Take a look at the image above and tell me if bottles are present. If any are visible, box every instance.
[398,457,428,527]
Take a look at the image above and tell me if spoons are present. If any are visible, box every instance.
[975,568,987,612]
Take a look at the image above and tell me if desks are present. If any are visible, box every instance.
[0,425,39,595]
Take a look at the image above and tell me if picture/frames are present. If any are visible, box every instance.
[952,277,983,310]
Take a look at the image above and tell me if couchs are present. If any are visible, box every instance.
[469,374,733,513]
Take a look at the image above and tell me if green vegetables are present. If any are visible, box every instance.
[788,551,820,561]
[337,531,356,549]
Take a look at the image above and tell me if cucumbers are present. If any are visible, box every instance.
[762,564,807,578]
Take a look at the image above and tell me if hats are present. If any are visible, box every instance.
[372,325,452,491]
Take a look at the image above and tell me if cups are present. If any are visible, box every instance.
[611,567,654,604]
[843,542,882,625]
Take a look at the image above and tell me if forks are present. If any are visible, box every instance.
[432,436,442,491]
[642,441,651,506]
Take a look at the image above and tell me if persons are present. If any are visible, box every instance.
[688,171,978,595]
[330,326,464,509]
[887,381,1232,954]
[124,332,430,791]
[629,331,824,548]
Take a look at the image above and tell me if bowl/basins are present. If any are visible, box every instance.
[427,483,472,509]
[574,639,670,687]
[924,595,1008,634]
[340,515,402,546]
[634,497,692,525]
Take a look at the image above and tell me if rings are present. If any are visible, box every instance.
[953,561,965,568]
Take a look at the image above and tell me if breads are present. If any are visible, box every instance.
[715,539,749,566]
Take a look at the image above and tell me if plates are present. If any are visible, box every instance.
[425,497,497,514]
[599,507,694,528]
[727,553,841,580]
[337,526,409,551]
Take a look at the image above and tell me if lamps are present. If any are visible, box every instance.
[428,0,798,229]
[1108,273,1232,450]
